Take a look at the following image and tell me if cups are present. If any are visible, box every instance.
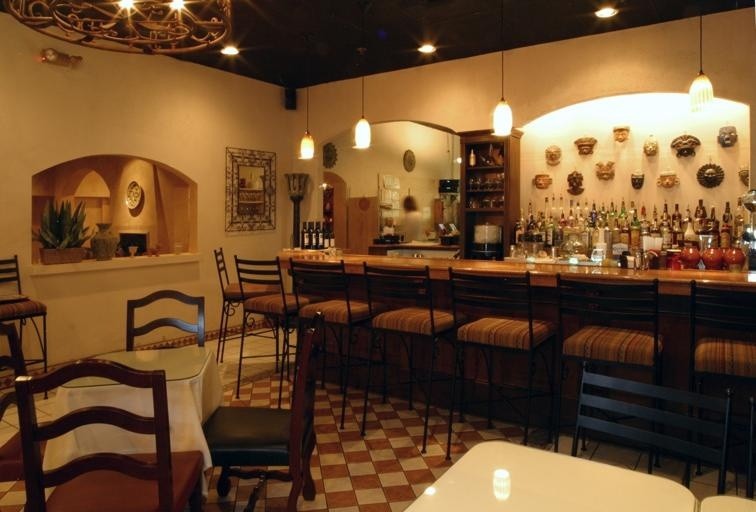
[129,246,137,257]
[591,249,605,261]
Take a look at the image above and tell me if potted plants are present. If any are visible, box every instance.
[36,199,93,265]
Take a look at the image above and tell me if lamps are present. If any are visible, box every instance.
[352,119,372,149]
[284,172,309,200]
[7,0,233,55]
[490,96,513,137]
[297,130,315,160]
[688,70,714,115]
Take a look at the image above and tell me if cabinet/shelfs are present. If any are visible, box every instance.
[460,129,524,261]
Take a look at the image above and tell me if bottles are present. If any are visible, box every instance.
[660,200,671,227]
[720,223,731,249]
[469,149,476,166]
[309,221,316,249]
[696,220,707,235]
[694,199,708,218]
[708,223,719,235]
[630,226,640,246]
[672,213,684,247]
[693,209,706,232]
[521,193,630,247]
[684,218,697,246]
[639,206,650,235]
[514,222,522,245]
[324,222,330,249]
[630,208,640,226]
[707,207,720,232]
[735,196,745,218]
[316,221,324,249]
[650,218,661,237]
[330,230,335,247]
[301,222,309,249]
[723,201,732,226]
[628,200,635,220]
[650,204,659,225]
[660,211,672,251]
[682,204,693,232]
[672,199,681,228]
[734,214,745,244]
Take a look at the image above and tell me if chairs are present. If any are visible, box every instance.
[0,255,49,400]
[288,256,380,430]
[201,311,324,512]
[358,260,454,453]
[14,357,204,512]
[442,265,559,454]
[682,278,756,497]
[235,254,309,408]
[125,289,205,351]
[552,271,670,474]
[212,246,281,365]
